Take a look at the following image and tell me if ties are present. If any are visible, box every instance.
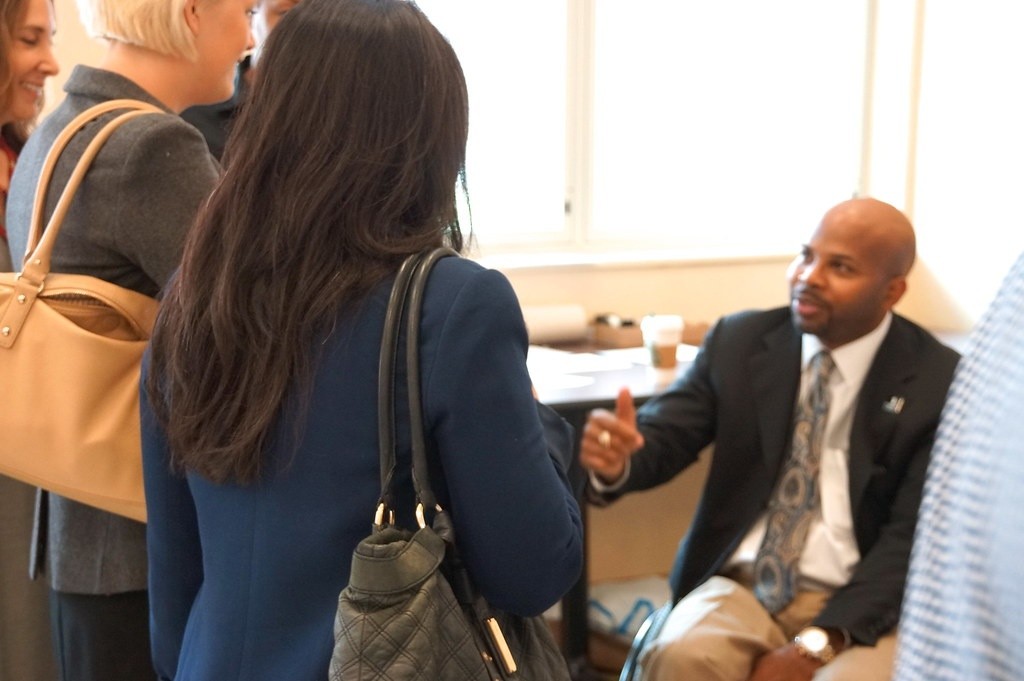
[753,350,836,616]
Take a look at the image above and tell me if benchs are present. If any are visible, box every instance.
[543,578,674,672]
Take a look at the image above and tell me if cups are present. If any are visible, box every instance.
[640,314,682,368]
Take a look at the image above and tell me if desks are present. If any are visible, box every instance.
[526,341,702,681]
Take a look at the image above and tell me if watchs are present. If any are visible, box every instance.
[794,624,835,664]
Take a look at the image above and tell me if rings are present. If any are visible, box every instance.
[598,428,612,449]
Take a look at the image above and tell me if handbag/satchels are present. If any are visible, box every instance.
[328,246,572,681]
[0,100,166,524]
[581,576,672,647]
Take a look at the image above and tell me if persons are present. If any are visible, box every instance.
[579,194,964,681]
[136,1,589,681]
[0,0,304,681]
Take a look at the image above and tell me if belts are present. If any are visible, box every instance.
[717,561,834,592]
